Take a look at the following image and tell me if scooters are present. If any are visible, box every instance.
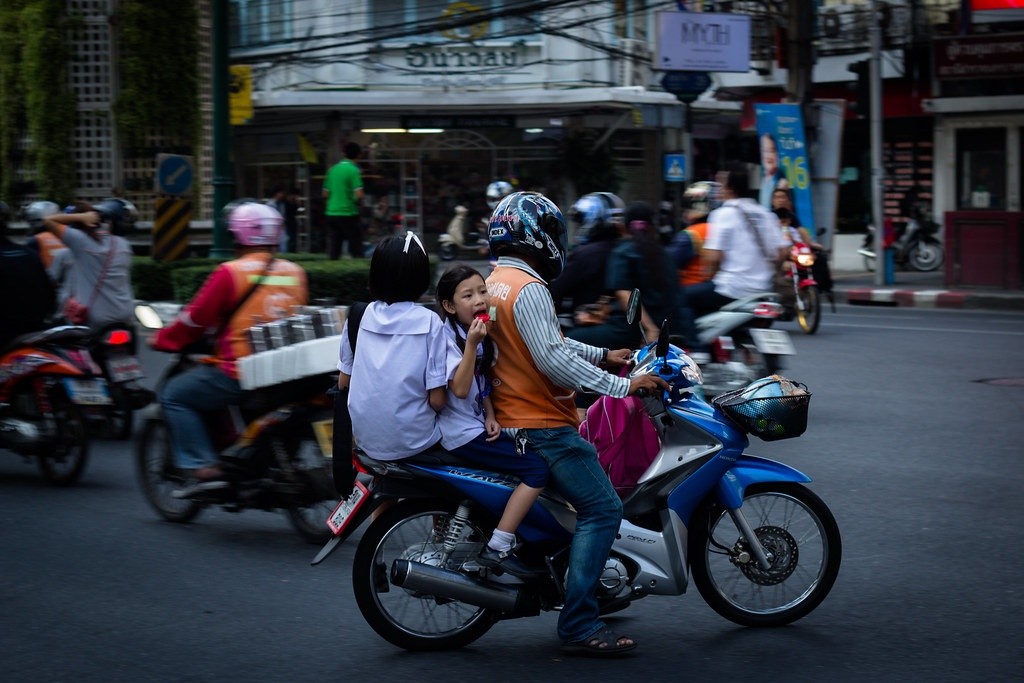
[437,204,491,261]
[692,290,798,382]
[834,199,945,273]
[0,320,161,488]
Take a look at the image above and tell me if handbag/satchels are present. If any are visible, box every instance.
[64,299,89,324]
[732,375,806,422]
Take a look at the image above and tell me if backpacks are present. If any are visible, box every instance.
[577,356,660,493]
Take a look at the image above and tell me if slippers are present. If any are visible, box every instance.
[171,472,229,497]
[561,623,638,654]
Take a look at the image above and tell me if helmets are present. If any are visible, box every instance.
[677,181,724,225]
[88,197,130,234]
[227,202,283,246]
[486,191,567,282]
[485,182,514,206]
[24,200,59,228]
[569,190,628,245]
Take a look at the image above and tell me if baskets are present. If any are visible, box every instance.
[920,221,939,233]
[713,374,812,442]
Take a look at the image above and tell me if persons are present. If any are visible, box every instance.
[759,133,783,209]
[266,187,300,252]
[438,264,550,577]
[148,199,308,499]
[321,141,368,260]
[483,192,672,653]
[558,167,821,350]
[0,194,135,360]
[336,230,448,593]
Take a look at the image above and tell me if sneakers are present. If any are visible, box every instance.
[476,543,537,579]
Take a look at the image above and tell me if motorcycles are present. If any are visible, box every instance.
[129,304,374,545]
[784,225,832,333]
[309,285,842,657]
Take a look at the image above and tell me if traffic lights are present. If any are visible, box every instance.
[846,59,870,120]
